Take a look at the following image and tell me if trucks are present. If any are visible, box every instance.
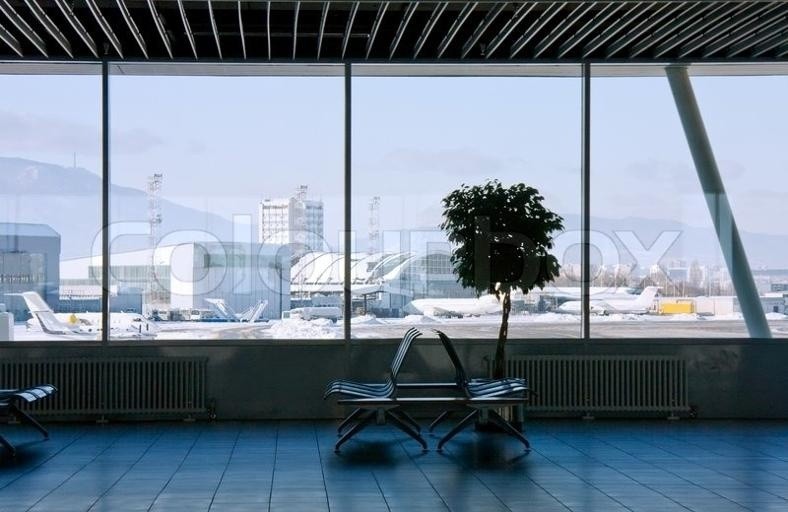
[288,307,341,324]
[119,306,212,321]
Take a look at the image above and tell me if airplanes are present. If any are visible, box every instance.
[3,291,160,338]
[554,282,664,317]
[396,287,514,317]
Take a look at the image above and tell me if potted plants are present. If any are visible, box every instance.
[438,178,565,438]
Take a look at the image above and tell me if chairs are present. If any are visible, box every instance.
[426,329,533,452]
[323,326,427,454]
[0,383,58,457]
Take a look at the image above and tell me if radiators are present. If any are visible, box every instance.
[0,356,217,425]
[485,352,691,422]
